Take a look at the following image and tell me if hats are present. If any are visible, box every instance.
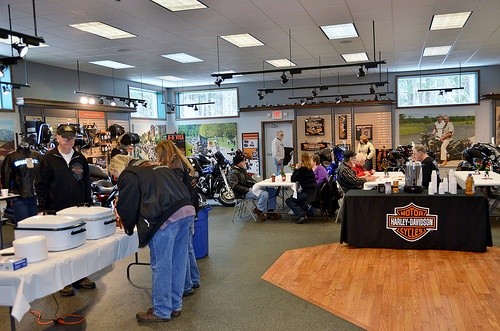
[233,154,245,165]
[344,150,357,158]
[57,125,76,138]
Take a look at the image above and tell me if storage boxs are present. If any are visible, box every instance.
[1,219,15,239]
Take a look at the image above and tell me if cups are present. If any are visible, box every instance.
[272,175,275,182]
[1,189,9,197]
[428,169,457,196]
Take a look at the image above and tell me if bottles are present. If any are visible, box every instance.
[465,174,476,194]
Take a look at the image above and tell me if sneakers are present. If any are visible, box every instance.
[73,277,96,288]
[60,286,76,295]
[267,211,281,219]
[253,208,266,220]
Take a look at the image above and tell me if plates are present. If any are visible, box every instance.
[12,235,48,263]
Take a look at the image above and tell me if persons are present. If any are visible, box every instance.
[230,131,439,224]
[109,141,196,321]
[431,115,454,166]
[154,140,200,296]
[2,141,43,226]
[35,125,98,295]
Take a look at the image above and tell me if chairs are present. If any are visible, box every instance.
[335,180,345,223]
[304,178,335,222]
[221,171,261,223]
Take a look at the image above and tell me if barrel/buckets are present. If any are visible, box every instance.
[404,161,426,192]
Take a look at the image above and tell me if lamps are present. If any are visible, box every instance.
[194,106,199,111]
[370,84,378,101]
[335,96,342,104]
[213,77,223,88]
[299,88,318,105]
[356,65,366,78]
[0,64,7,77]
[2,83,12,95]
[11,43,28,57]
[78,95,148,110]
[438,90,444,96]
[257,89,265,101]
[170,104,176,111]
[279,71,288,85]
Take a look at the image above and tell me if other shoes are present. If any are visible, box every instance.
[297,215,308,224]
[148,308,179,316]
[136,311,168,322]
[183,290,193,296]
[192,284,199,288]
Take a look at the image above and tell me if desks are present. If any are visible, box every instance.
[0,223,151,331]
[340,189,494,253]
[362,170,407,190]
[250,172,302,223]
[454,169,500,219]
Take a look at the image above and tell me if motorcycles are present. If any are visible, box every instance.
[420,133,473,162]
[88,164,119,207]
[187,148,236,206]
